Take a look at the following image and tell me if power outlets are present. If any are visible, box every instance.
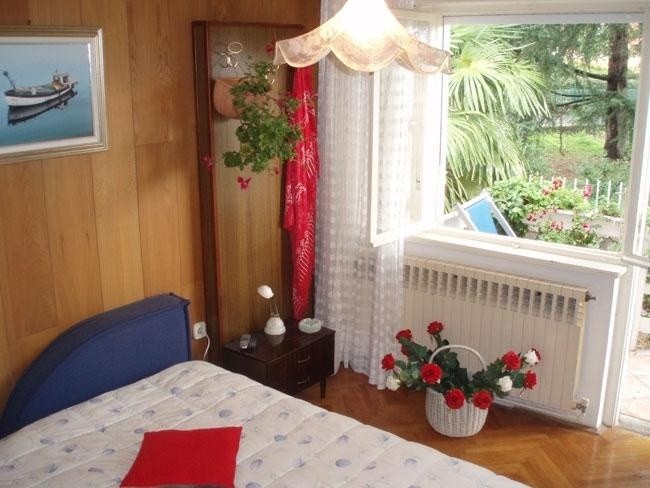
[191,319,209,341]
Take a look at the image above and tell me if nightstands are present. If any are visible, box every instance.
[216,313,338,403]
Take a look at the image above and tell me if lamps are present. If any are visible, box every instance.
[255,283,287,336]
[272,0,455,77]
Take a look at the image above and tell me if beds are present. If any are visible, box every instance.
[1,291,540,488]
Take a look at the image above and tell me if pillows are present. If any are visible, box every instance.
[116,422,243,488]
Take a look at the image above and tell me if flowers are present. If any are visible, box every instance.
[379,319,540,410]
[202,39,303,193]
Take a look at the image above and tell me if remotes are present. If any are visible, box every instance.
[238,334,251,349]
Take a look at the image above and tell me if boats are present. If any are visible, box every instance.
[5,90,77,126]
[0,61,79,107]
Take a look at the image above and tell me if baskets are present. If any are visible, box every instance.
[425,345,490,438]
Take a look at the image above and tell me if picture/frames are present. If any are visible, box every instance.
[0,22,111,168]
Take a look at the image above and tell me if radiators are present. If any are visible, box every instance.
[343,244,598,420]
